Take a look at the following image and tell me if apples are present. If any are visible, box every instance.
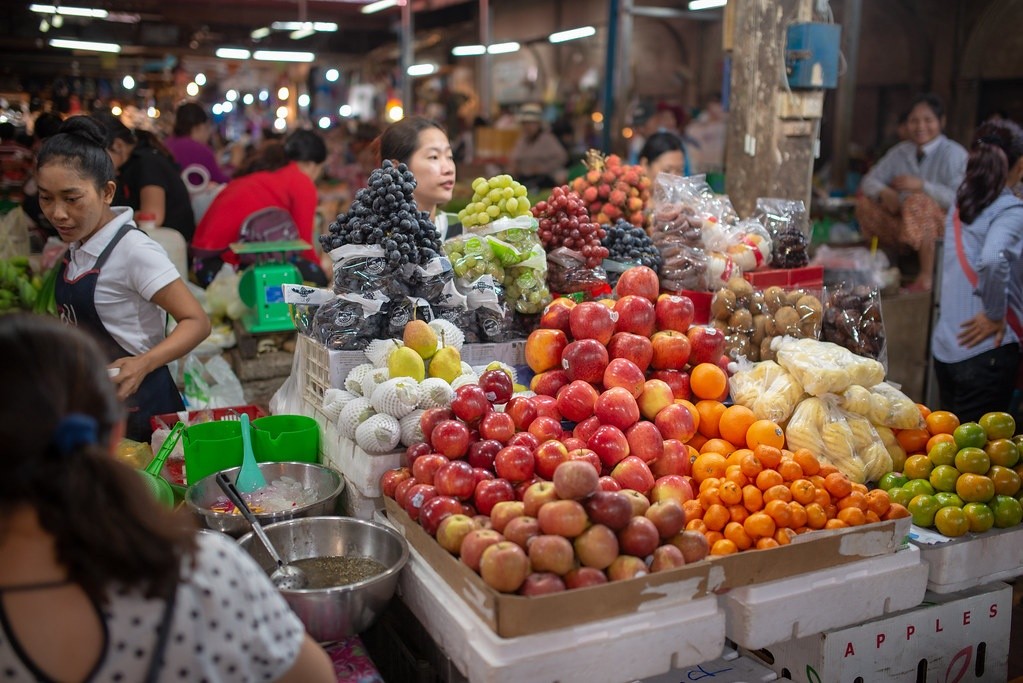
[381,265,730,599]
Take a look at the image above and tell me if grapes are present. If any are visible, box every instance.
[294,158,661,348]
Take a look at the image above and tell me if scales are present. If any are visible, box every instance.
[228,240,312,333]
[813,198,856,242]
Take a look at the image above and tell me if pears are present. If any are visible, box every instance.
[342,300,530,453]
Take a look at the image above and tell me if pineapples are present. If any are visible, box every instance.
[728,339,922,484]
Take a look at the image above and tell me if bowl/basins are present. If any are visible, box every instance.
[236,516,409,642]
[185,462,345,541]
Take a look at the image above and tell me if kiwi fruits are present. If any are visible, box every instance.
[709,277,824,362]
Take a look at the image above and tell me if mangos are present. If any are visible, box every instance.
[0,256,50,315]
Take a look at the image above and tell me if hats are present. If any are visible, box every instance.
[516,103,543,123]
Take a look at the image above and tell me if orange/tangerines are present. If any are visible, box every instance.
[670,362,909,555]
[874,405,1023,538]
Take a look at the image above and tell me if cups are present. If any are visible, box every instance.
[184,420,254,486]
[250,415,317,464]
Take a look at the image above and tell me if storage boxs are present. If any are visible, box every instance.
[271,266,1023,683]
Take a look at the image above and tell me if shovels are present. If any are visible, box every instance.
[235,412,267,494]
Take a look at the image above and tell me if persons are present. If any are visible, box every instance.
[240,127,281,151]
[930,113,1023,439]
[0,103,62,252]
[191,128,327,288]
[37,115,212,444]
[161,101,231,187]
[0,310,337,683]
[314,115,462,243]
[859,94,969,213]
[87,109,197,245]
[629,91,728,197]
[511,103,570,188]
